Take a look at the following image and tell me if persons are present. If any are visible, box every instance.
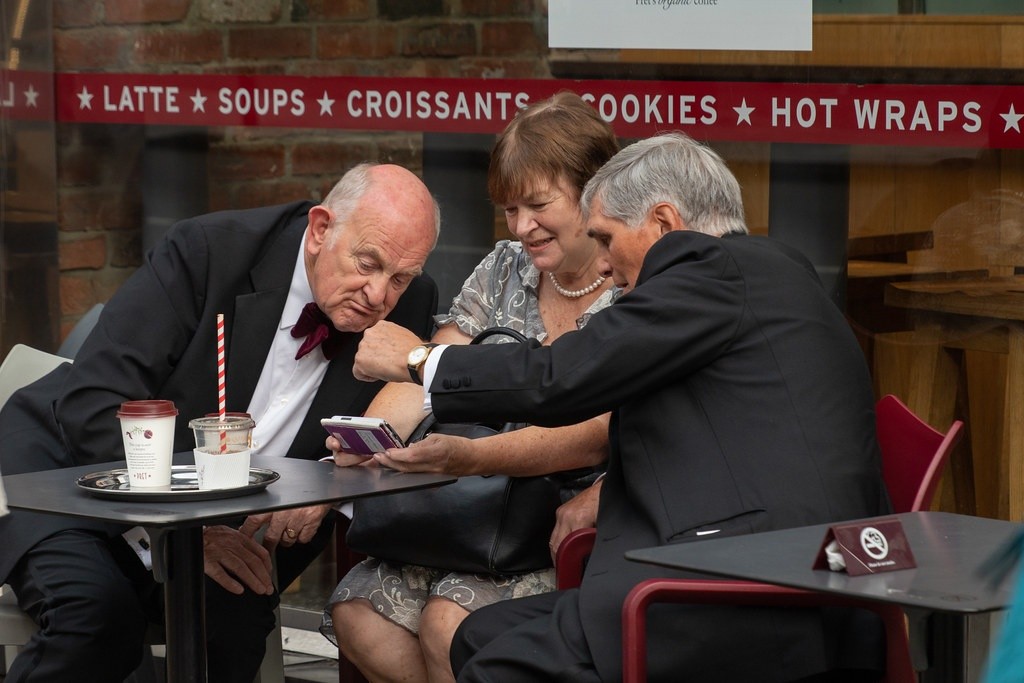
[353,133,897,683]
[326,93,622,683]
[0,163,440,683]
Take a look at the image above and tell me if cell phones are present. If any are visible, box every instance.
[321,415,406,455]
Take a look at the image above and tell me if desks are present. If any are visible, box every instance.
[624,510,1024,683]
[0,418,458,683]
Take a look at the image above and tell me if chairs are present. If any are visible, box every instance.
[0,300,962,682]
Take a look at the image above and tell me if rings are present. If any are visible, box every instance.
[285,527,300,538]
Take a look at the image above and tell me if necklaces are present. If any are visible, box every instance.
[546,268,605,299]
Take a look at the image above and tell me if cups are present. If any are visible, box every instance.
[189,417,256,490]
[202,413,251,449]
[193,445,249,489]
[117,400,178,488]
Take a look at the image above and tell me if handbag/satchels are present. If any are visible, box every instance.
[346,326,563,578]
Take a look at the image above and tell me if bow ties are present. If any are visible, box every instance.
[290,303,333,361]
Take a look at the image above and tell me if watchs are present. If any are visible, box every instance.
[407,342,439,386]
